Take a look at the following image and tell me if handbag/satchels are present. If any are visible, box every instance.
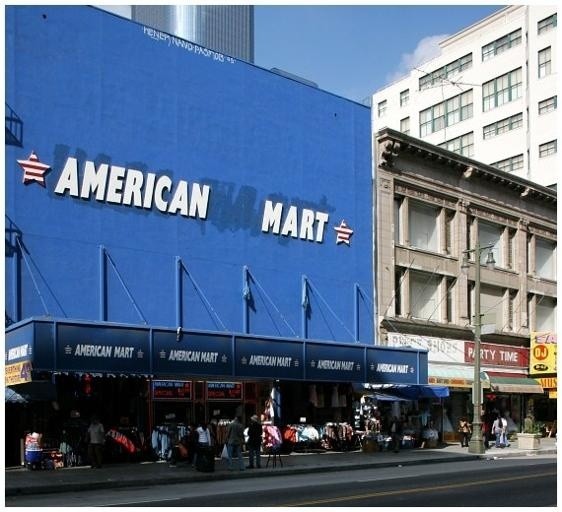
[500,434,507,445]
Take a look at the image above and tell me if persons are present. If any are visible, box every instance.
[223,416,246,471]
[386,414,402,452]
[92,419,105,469]
[246,414,263,469]
[458,416,473,447]
[520,412,533,432]
[191,421,212,470]
[87,416,98,468]
[489,415,508,449]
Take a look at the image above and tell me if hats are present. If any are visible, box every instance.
[250,415,258,422]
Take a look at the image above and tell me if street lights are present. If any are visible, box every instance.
[460,241,497,453]
